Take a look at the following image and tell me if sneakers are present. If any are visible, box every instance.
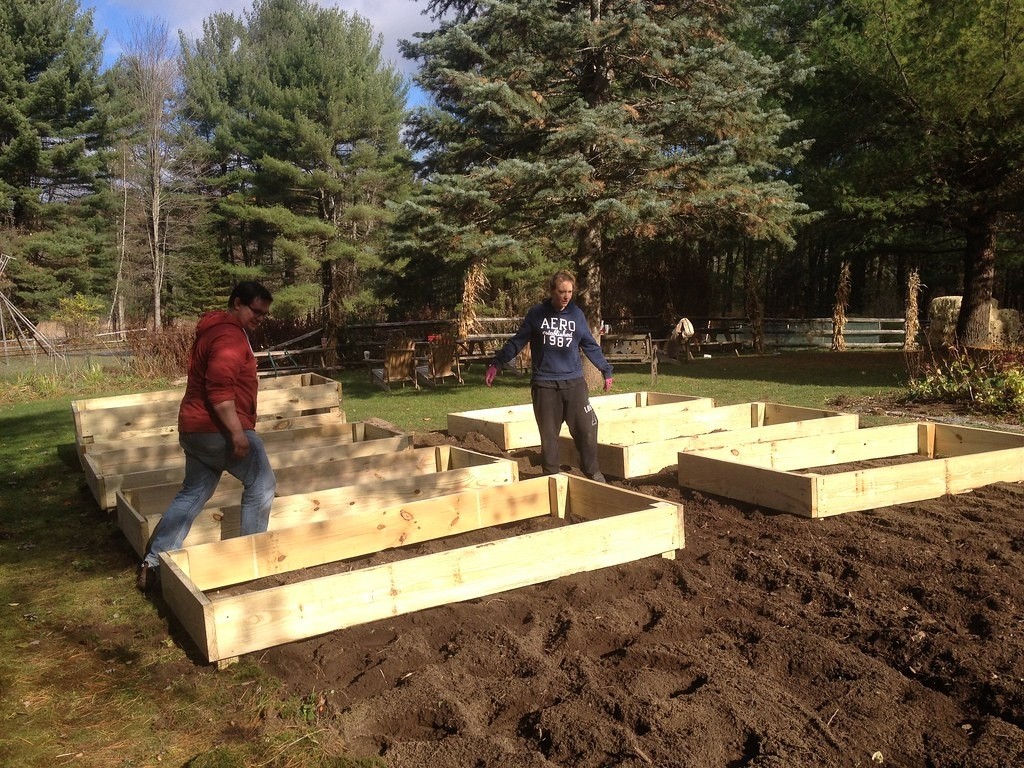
[138,562,162,592]
[591,470,606,484]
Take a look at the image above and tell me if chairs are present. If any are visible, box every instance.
[370,337,421,393]
[413,321,465,387]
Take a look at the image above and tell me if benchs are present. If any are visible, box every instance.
[453,355,496,360]
[602,333,659,386]
[689,341,747,345]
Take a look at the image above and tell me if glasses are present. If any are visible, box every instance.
[248,305,270,320]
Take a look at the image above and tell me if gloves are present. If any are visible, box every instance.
[603,377,613,393]
[485,364,497,388]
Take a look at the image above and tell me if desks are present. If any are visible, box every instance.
[459,333,528,379]
[677,328,745,358]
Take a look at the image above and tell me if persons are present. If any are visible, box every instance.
[485,270,613,484]
[138,281,277,588]
